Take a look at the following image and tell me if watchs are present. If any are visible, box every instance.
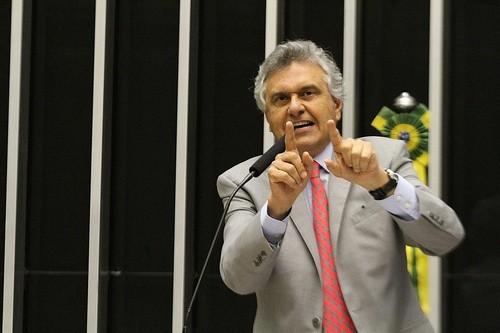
[369,168,398,200]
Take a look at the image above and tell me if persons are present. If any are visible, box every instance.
[216,39,465,333]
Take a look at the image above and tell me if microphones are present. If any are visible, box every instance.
[248,133,288,178]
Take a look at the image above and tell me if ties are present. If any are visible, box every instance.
[304,162,356,333]
